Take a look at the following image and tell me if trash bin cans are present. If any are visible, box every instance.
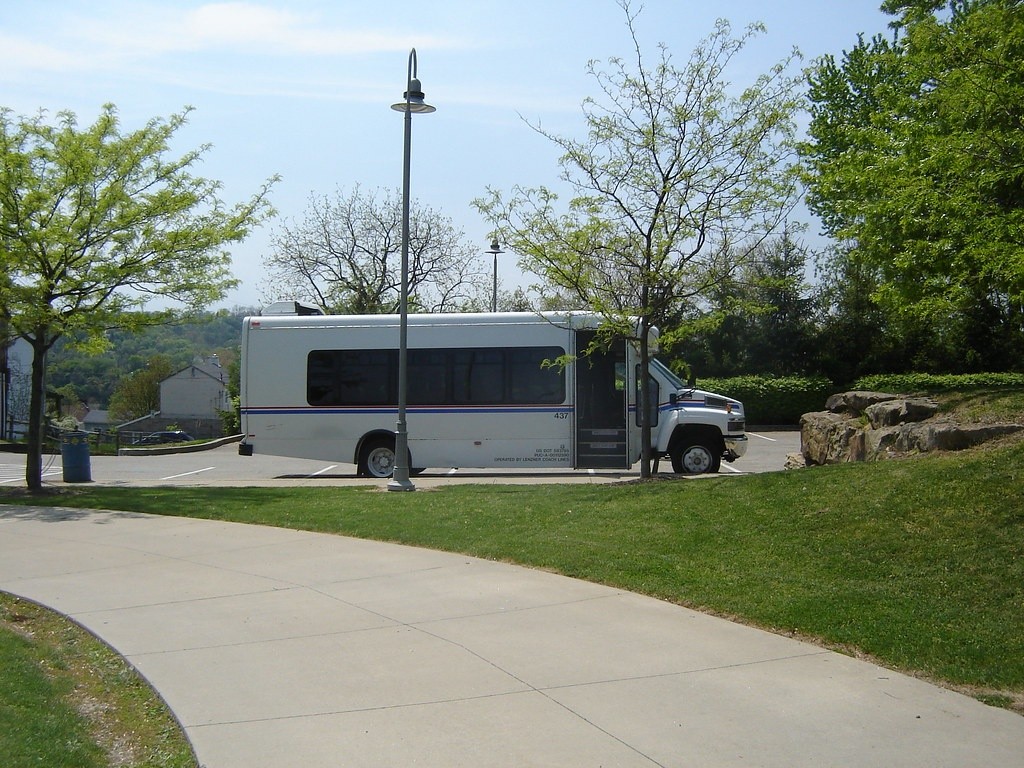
[58,431,96,483]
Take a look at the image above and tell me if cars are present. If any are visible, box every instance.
[132,431,194,445]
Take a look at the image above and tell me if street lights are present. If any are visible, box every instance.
[484,229,506,312]
[389,46,438,493]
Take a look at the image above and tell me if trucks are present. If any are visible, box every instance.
[237,301,749,475]
